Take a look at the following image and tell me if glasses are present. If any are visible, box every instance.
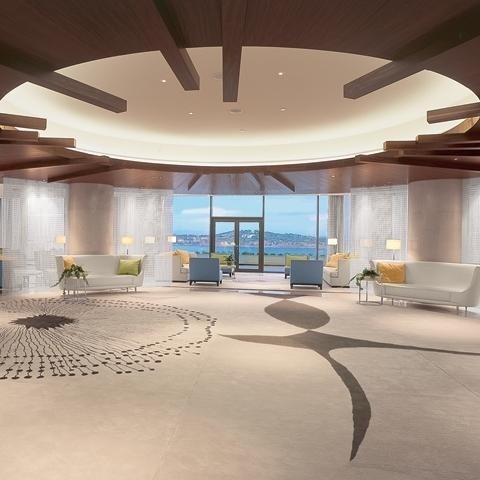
[384,236,404,262]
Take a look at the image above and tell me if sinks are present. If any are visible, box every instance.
[54,249,235,301]
[284,250,480,317]
